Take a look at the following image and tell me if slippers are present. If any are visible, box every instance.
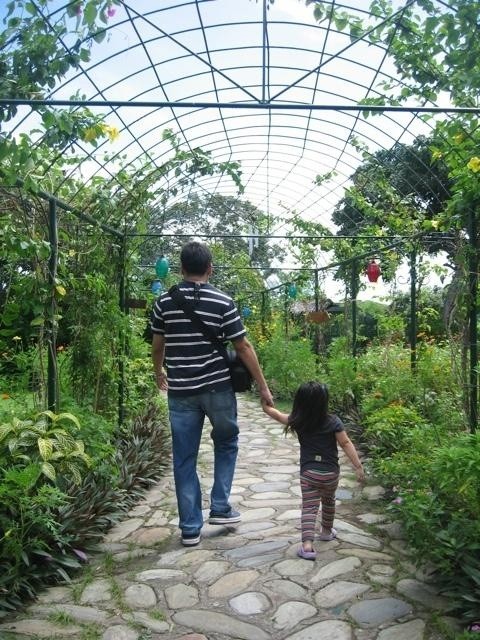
[320,528,336,540]
[297,544,316,559]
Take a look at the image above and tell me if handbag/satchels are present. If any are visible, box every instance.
[227,350,255,392]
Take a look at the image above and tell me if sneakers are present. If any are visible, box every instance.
[208,511,241,524]
[182,528,201,544]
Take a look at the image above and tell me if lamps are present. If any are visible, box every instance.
[367,259,380,282]
[156,253,168,278]
[289,287,297,297]
[151,280,162,291]
[243,306,250,318]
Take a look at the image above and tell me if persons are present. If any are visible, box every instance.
[145,241,275,547]
[261,380,366,560]
